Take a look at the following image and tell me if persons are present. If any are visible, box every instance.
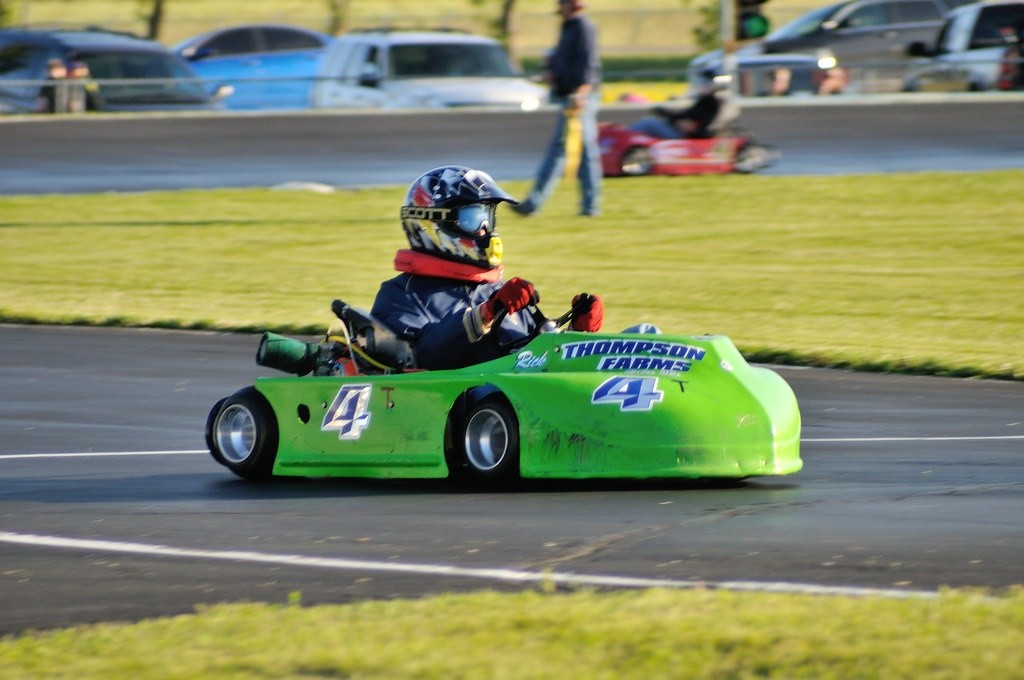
[506,0,605,218]
[627,67,727,140]
[61,60,107,108]
[360,165,606,374]
[30,47,68,113]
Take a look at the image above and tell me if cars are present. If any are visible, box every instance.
[688,0,949,96]
[903,0,1024,92]
[0,27,235,113]
[171,24,330,109]
[309,27,548,108]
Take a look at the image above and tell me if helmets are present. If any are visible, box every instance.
[401,163,518,270]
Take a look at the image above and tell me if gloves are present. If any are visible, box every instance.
[572,294,604,332]
[480,277,538,327]
